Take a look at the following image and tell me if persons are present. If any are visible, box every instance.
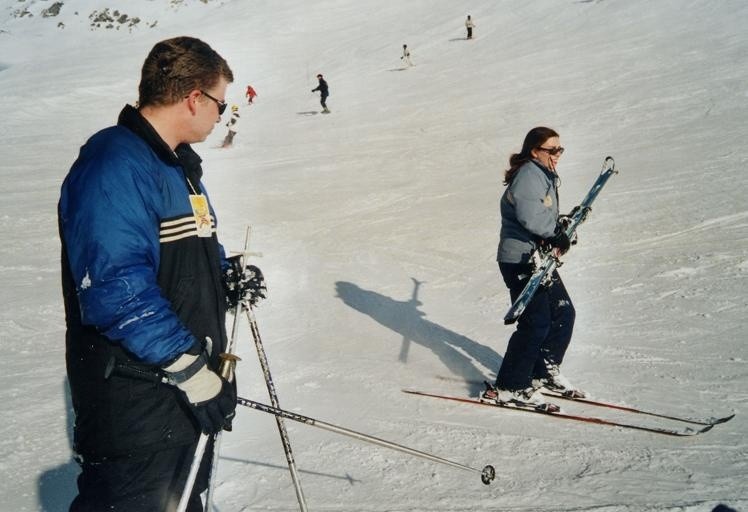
[482,127,594,415]
[217,104,242,149]
[309,73,331,114]
[56,35,269,511]
[400,42,415,68]
[244,84,258,106]
[462,15,476,40]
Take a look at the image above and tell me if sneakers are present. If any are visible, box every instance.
[479,376,584,412]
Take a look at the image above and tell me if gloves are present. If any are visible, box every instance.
[161,343,237,434]
[552,233,570,253]
[226,265,267,313]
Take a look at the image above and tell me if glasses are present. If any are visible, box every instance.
[540,147,564,154]
[200,90,226,114]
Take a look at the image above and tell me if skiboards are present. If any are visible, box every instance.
[401,376,736,437]
[504,156,618,326]
